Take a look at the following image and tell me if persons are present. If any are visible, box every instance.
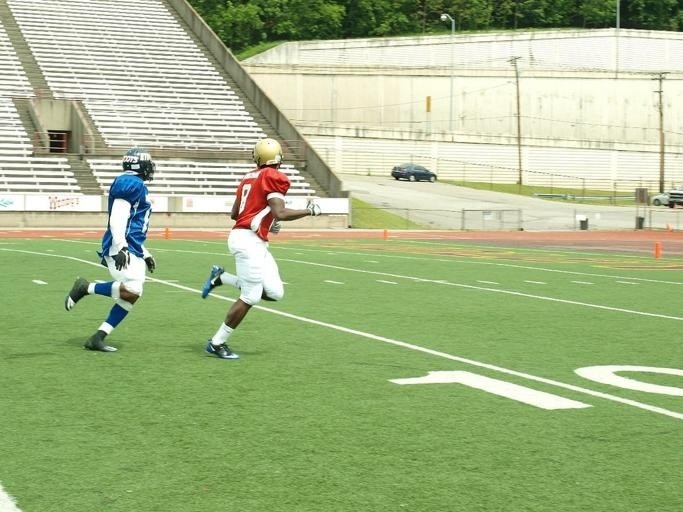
[198,134,321,360]
[63,147,161,352]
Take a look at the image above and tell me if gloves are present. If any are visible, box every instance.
[270,219,281,233]
[114,248,131,271]
[306,198,325,216]
[144,257,158,273]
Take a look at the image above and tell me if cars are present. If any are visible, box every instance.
[392,163,437,182]
[651,187,683,208]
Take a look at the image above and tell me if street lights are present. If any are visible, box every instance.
[440,13,455,132]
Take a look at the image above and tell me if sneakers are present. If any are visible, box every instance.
[82,337,119,353]
[201,265,225,298]
[65,277,89,312]
[206,343,240,360]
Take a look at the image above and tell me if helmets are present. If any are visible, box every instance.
[122,147,156,181]
[252,137,284,168]
